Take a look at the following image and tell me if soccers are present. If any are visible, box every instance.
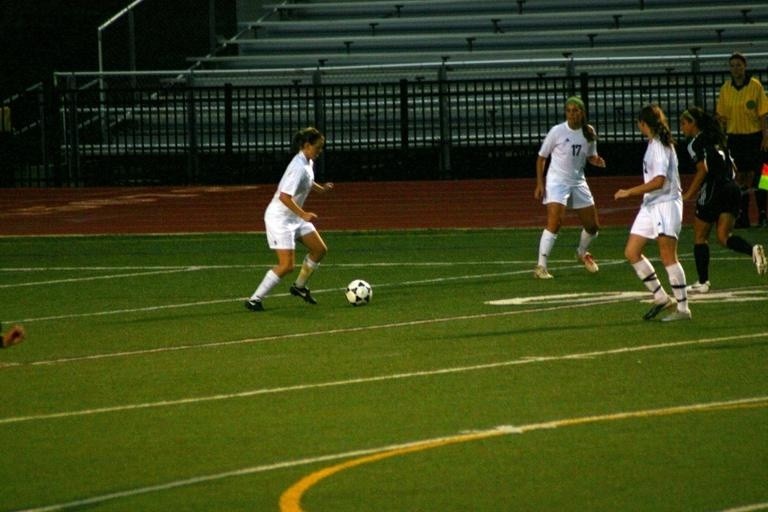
[344,279,373,307]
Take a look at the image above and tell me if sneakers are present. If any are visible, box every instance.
[758,214,768,228]
[641,295,672,321]
[686,279,712,294]
[244,299,265,313]
[573,248,599,274]
[661,309,692,322]
[533,264,554,281]
[749,243,768,276]
[733,215,752,229]
[289,283,317,305]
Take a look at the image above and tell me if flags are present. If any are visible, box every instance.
[757,163,768,192]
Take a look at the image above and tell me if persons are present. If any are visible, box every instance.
[677,105,767,296]
[613,102,694,324]
[716,55,768,227]
[534,96,606,279]
[0,323,27,349]
[241,125,336,312]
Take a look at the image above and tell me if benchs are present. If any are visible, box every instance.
[103,1,768,136]
